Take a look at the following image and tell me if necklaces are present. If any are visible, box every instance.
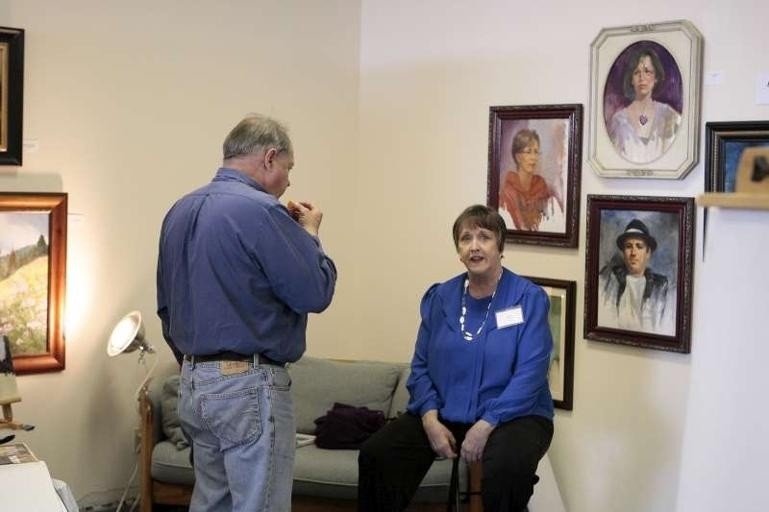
[459,269,505,342]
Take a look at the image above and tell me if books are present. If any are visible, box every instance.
[0,441,68,511]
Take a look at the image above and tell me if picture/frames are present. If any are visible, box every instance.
[1,25,26,168]
[697,118,768,209]
[587,18,706,184]
[485,101,585,250]
[0,190,71,379]
[579,192,698,357]
[518,273,578,415]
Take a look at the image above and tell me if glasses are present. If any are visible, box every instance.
[513,147,543,154]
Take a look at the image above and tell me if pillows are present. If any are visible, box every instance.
[389,366,412,418]
[158,376,192,451]
[281,356,401,437]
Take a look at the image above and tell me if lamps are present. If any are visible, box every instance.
[105,308,162,511]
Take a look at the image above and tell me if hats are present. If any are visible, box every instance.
[616,219,656,249]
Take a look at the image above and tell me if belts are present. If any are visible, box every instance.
[181,350,287,372]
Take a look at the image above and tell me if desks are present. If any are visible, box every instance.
[0,439,68,512]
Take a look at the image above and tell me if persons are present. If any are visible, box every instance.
[497,130,567,234]
[156,117,338,512]
[612,47,681,164]
[596,218,675,337]
[358,204,556,511]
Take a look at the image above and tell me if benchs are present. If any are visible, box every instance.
[133,353,485,511]
